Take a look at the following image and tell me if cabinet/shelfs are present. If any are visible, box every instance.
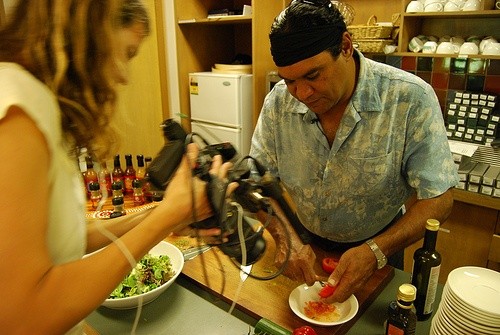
[173,0,285,152]
[399,0,500,59]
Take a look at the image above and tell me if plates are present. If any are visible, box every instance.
[428,265,500,335]
[289,281,359,326]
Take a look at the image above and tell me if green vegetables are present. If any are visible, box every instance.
[107,251,177,299]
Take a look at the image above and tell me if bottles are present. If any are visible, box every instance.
[85,155,98,202]
[113,154,123,195]
[98,156,113,197]
[123,154,134,196]
[385,284,417,335]
[135,155,147,194]
[409,219,441,321]
[144,157,154,193]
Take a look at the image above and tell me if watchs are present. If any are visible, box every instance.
[365,238,388,270]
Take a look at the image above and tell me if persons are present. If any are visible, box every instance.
[246,0,458,305]
[0,0,239,335]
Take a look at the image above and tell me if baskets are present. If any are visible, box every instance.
[353,39,394,53]
[348,14,393,38]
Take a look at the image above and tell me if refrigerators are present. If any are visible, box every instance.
[187,72,253,156]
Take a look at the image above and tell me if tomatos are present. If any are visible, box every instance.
[291,326,317,335]
[318,283,337,298]
[322,258,342,273]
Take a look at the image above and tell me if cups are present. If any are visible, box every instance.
[436,42,460,54]
[417,35,497,53]
[409,37,423,53]
[459,43,479,54]
[442,0,464,12]
[423,0,444,13]
[482,43,500,55]
[405,1,425,12]
[462,0,481,11]
[422,41,437,53]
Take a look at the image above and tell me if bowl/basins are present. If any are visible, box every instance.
[82,240,184,310]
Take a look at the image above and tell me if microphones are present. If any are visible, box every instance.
[254,159,312,245]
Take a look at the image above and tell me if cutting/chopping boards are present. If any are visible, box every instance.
[163,221,394,335]
